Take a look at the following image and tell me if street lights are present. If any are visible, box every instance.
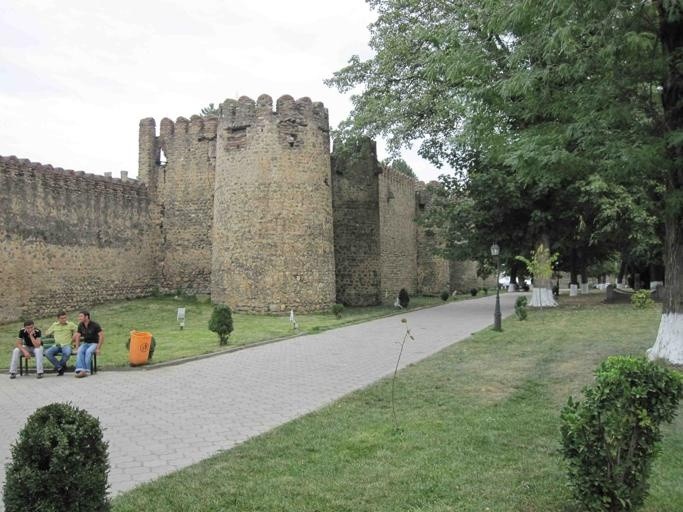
[491,241,502,330]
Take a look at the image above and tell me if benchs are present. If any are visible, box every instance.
[20,335,96,375]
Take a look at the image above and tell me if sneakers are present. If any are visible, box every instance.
[36,372,43,378]
[75,370,89,378]
[10,372,17,379]
[56,367,64,375]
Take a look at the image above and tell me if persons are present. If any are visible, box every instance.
[74,310,104,378]
[44,310,79,376]
[9,319,44,378]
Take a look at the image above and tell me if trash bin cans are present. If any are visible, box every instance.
[129,330,153,366]
